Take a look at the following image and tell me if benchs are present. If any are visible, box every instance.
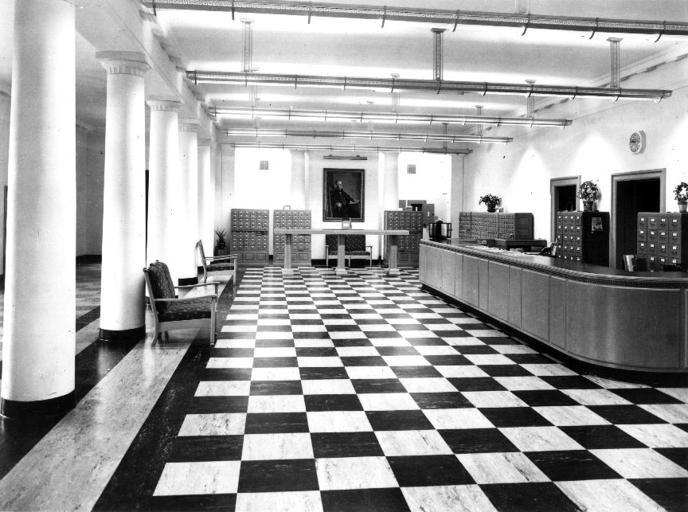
[325,229,373,268]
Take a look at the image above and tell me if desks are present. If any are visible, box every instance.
[276,227,410,276]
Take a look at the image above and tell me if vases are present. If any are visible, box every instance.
[582,199,594,212]
[488,203,496,212]
[677,200,686,212]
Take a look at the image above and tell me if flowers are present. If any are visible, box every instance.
[575,181,601,201]
[673,183,688,202]
[480,194,501,206]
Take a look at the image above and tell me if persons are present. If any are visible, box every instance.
[330,180,360,218]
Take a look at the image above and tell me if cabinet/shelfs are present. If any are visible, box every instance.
[557,211,610,265]
[635,213,688,266]
[383,211,422,268]
[459,213,533,249]
[229,208,310,266]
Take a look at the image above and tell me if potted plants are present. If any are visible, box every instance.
[217,229,227,248]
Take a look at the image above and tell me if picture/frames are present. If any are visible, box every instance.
[323,168,364,222]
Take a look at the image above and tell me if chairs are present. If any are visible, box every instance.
[143,240,237,348]
